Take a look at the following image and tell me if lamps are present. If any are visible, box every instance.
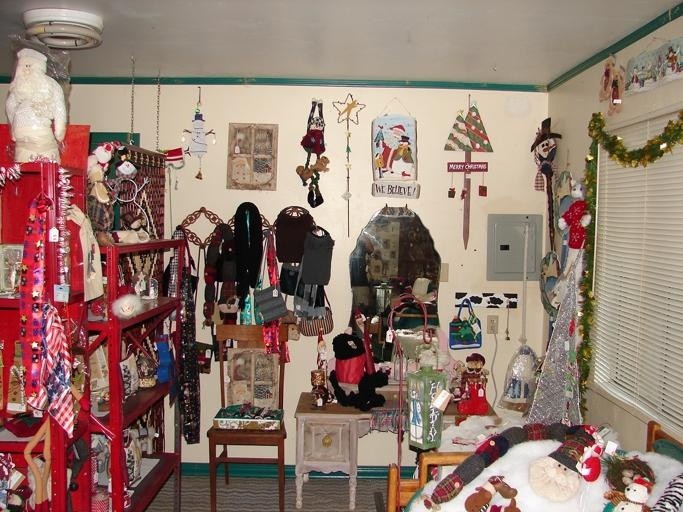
[22,8,103,52]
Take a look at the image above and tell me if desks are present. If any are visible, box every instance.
[295,393,500,510]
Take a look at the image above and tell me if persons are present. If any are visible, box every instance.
[349,234,373,306]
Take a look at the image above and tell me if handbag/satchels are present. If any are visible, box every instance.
[254,232,334,337]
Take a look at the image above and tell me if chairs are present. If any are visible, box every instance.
[207,323,286,510]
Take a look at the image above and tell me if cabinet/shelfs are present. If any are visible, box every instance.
[0,125,186,512]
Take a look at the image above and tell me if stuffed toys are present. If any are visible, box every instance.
[600,455,654,512]
[6,48,67,165]
[614,477,654,512]
[529,425,605,502]
[558,179,591,250]
[465,475,521,512]
